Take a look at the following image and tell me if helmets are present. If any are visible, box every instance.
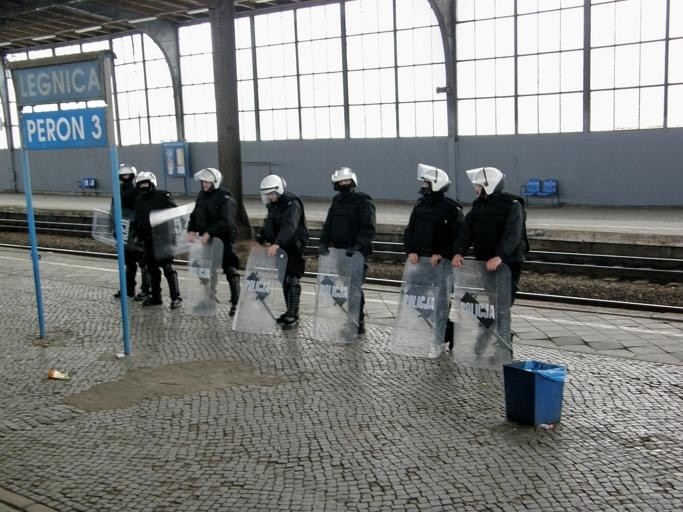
[331,167,358,187]
[194,167,222,189]
[119,164,157,187]
[418,164,449,192]
[466,167,503,195]
[260,175,284,195]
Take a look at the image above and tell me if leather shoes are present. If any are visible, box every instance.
[445,319,454,348]
[276,313,299,330]
[115,291,180,308]
[230,305,236,315]
[340,325,365,335]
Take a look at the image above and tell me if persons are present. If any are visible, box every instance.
[135,171,185,309]
[107,164,152,301]
[319,167,375,333]
[404,164,465,348]
[187,167,241,316]
[256,174,309,329]
[451,167,529,360]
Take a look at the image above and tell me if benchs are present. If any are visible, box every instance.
[74,177,98,196]
[520,178,560,208]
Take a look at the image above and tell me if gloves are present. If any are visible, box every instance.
[347,243,367,257]
[319,239,334,255]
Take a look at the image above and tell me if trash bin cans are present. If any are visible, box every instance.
[503,361,566,427]
